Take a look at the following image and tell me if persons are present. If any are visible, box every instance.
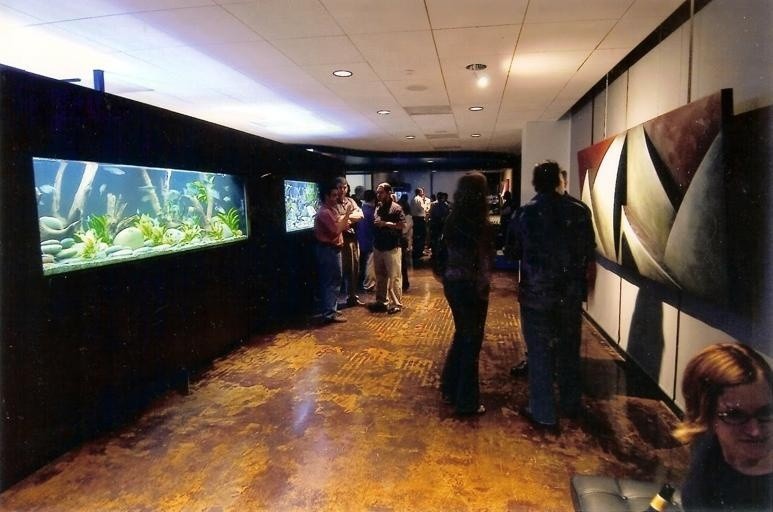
[410,187,451,266]
[501,191,512,253]
[669,341,772,512]
[314,177,413,323]
[433,172,489,415]
[508,159,598,428]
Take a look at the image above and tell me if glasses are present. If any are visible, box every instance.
[709,403,772,427]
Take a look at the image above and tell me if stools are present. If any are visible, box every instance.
[570,474,684,512]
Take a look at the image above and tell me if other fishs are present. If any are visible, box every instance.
[35,187,45,206]
[136,179,245,228]
[99,183,107,196]
[40,184,57,194]
[103,167,126,176]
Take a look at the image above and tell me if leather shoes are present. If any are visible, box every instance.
[511,359,528,376]
[519,402,556,428]
[327,294,401,322]
